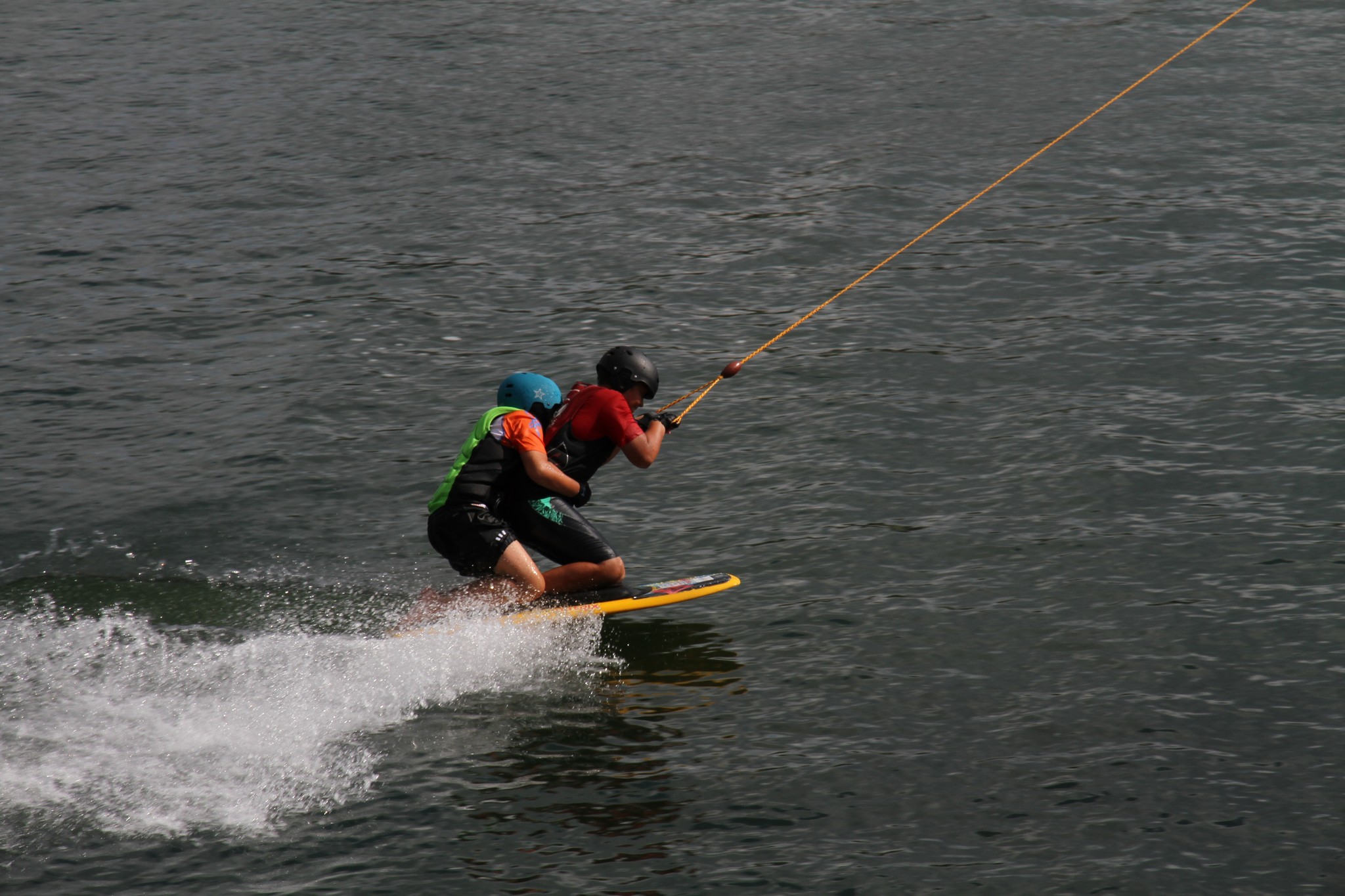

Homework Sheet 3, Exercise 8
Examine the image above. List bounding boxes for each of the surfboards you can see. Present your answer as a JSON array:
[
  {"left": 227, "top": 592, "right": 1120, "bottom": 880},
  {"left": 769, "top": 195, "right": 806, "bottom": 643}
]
[{"left": 387, "top": 571, "right": 743, "bottom": 643}]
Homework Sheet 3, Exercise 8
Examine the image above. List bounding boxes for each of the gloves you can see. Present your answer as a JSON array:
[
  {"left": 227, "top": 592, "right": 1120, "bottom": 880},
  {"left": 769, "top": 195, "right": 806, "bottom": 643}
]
[{"left": 569, "top": 482, "right": 593, "bottom": 508}]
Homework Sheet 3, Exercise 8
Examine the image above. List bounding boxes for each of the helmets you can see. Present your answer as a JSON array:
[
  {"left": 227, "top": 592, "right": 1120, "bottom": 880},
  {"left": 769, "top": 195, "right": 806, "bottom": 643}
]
[
  {"left": 600, "top": 346, "right": 660, "bottom": 401},
  {"left": 497, "top": 372, "right": 563, "bottom": 426}
]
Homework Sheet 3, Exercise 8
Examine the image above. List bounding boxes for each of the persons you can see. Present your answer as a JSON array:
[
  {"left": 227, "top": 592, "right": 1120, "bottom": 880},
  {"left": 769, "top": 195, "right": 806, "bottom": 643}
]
[
  {"left": 402, "top": 370, "right": 593, "bottom": 628},
  {"left": 499, "top": 346, "right": 679, "bottom": 594}
]
